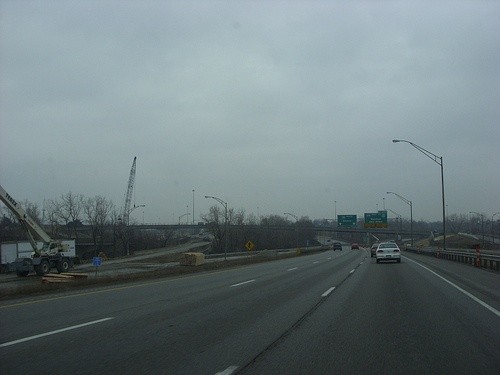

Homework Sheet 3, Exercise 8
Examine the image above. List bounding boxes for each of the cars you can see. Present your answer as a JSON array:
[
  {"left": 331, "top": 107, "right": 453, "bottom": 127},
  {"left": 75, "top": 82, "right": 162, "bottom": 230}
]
[
  {"left": 376, "top": 242, "right": 402, "bottom": 263},
  {"left": 352, "top": 243, "right": 360, "bottom": 250},
  {"left": 371, "top": 243, "right": 379, "bottom": 257}
]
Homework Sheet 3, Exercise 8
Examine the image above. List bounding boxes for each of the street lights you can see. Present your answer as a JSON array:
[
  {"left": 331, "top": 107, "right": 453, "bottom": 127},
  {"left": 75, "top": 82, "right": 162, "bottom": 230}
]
[
  {"left": 178, "top": 213, "right": 191, "bottom": 241},
  {"left": 192, "top": 189, "right": 195, "bottom": 225},
  {"left": 334, "top": 201, "right": 337, "bottom": 219},
  {"left": 386, "top": 191, "right": 414, "bottom": 247},
  {"left": 469, "top": 211, "right": 485, "bottom": 248},
  {"left": 283, "top": 213, "right": 299, "bottom": 257},
  {"left": 384, "top": 208, "right": 403, "bottom": 230},
  {"left": 383, "top": 198, "right": 386, "bottom": 210},
  {"left": 129, "top": 205, "right": 146, "bottom": 214},
  {"left": 393, "top": 139, "right": 446, "bottom": 250},
  {"left": 492, "top": 212, "right": 500, "bottom": 244},
  {"left": 205, "top": 195, "right": 228, "bottom": 260}
]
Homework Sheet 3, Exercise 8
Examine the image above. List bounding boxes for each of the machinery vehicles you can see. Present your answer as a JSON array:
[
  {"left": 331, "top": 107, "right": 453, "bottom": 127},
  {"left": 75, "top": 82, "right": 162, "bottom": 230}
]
[{"left": 0, "top": 186, "right": 74, "bottom": 276}]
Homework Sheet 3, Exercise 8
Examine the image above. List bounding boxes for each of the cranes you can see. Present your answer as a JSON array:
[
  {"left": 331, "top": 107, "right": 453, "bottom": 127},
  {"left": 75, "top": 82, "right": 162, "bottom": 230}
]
[{"left": 123, "top": 157, "right": 137, "bottom": 224}]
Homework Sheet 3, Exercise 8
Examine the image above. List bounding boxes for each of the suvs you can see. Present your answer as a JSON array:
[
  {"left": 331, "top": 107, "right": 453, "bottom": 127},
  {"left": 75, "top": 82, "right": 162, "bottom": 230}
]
[{"left": 333, "top": 242, "right": 343, "bottom": 251}]
